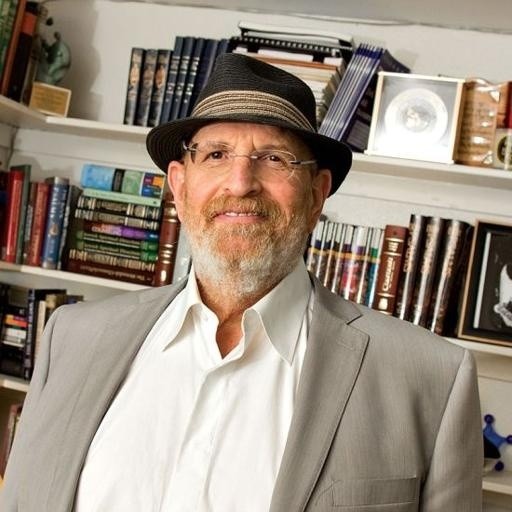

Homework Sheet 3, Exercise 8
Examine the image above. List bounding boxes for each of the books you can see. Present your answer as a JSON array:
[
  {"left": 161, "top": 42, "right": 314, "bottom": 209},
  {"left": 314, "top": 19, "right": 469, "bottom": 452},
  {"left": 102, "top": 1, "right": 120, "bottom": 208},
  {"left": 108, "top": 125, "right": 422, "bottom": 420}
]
[{"left": 0, "top": 403, "right": 23, "bottom": 478}]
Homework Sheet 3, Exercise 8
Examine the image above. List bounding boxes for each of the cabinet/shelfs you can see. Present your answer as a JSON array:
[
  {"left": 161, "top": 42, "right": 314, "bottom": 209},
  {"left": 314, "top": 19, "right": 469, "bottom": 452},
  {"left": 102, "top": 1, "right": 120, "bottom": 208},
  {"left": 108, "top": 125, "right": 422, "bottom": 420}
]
[{"left": 0, "top": 94, "right": 512, "bottom": 498}]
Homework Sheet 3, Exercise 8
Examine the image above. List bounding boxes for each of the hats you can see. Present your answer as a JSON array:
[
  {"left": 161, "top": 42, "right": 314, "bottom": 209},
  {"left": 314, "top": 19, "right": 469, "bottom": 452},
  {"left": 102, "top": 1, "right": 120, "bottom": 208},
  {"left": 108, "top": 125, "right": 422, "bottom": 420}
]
[{"left": 145, "top": 52, "right": 353, "bottom": 198}]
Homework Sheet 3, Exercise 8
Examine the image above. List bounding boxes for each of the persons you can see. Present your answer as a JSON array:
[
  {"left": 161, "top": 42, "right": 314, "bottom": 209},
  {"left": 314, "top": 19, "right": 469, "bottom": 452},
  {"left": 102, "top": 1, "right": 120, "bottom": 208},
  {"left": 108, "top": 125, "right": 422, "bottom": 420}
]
[{"left": 1, "top": 51, "right": 484, "bottom": 512}]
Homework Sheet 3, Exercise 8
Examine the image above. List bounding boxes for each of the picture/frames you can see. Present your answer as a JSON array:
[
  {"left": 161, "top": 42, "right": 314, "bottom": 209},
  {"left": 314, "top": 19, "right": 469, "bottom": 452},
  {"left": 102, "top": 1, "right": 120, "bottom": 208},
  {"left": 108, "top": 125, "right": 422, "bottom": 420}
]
[
  {"left": 363, "top": 71, "right": 468, "bottom": 164},
  {"left": 458, "top": 217, "right": 512, "bottom": 348}
]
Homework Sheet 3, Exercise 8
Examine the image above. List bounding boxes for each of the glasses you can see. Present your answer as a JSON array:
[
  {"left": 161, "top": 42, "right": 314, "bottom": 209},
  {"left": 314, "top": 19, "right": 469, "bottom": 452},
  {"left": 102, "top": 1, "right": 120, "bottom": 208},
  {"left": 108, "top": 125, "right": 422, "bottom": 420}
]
[{"left": 182, "top": 139, "right": 317, "bottom": 183}]
